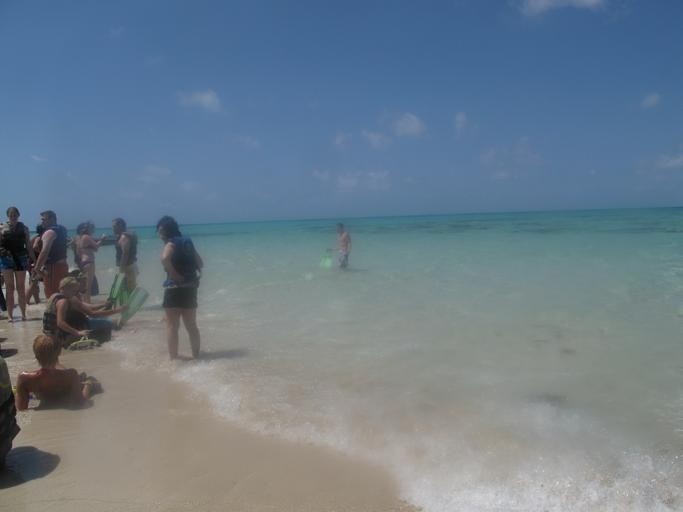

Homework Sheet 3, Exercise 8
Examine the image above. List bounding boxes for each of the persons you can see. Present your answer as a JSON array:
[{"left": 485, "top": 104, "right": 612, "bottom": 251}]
[{"left": 335, "top": 224, "right": 350, "bottom": 268}]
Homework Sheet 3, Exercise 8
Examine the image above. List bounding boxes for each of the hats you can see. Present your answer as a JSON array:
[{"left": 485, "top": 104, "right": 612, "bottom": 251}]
[{"left": 74, "top": 272, "right": 88, "bottom": 279}]
